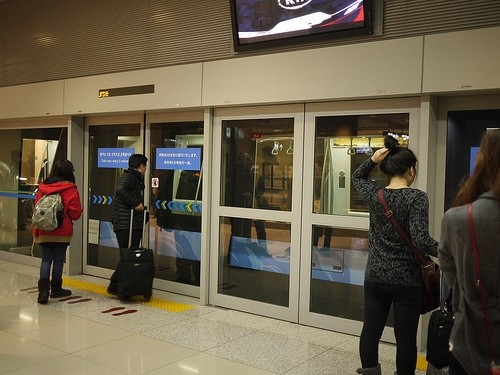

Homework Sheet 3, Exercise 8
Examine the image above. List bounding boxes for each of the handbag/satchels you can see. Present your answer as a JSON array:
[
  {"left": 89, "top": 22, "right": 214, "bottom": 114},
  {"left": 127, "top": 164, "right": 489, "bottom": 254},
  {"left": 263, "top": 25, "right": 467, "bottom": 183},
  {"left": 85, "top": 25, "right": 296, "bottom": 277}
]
[
  {"left": 427, "top": 310, "right": 454, "bottom": 369},
  {"left": 488, "top": 360, "right": 500, "bottom": 375},
  {"left": 424, "top": 255, "right": 440, "bottom": 294}
]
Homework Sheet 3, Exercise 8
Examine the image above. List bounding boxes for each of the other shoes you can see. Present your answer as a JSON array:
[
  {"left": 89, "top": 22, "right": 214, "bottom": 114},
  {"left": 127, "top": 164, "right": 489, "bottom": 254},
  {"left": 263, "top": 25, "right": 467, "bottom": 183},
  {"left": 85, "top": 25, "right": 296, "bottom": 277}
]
[
  {"left": 107, "top": 283, "right": 117, "bottom": 295},
  {"left": 257, "top": 247, "right": 272, "bottom": 258},
  {"left": 286, "top": 247, "right": 290, "bottom": 252},
  {"left": 319, "top": 247, "right": 330, "bottom": 254}
]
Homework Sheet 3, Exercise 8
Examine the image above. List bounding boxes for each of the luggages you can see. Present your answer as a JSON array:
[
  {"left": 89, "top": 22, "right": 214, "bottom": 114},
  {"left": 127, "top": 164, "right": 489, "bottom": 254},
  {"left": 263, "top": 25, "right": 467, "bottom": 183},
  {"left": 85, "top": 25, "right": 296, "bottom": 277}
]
[
  {"left": 117, "top": 206, "right": 154, "bottom": 302},
  {"left": 227, "top": 193, "right": 254, "bottom": 269}
]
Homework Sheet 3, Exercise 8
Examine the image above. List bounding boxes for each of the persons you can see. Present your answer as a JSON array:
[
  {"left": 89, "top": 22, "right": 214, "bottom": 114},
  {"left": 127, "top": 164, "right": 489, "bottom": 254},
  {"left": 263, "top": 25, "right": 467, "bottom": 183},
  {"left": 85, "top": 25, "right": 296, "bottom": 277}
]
[
  {"left": 440, "top": 130, "right": 500, "bottom": 375},
  {"left": 33, "top": 159, "right": 81, "bottom": 303},
  {"left": 157, "top": 145, "right": 332, "bottom": 286},
  {"left": 107, "top": 154, "right": 148, "bottom": 295},
  {"left": 3, "top": 149, "right": 35, "bottom": 233},
  {"left": 352, "top": 136, "right": 439, "bottom": 375}
]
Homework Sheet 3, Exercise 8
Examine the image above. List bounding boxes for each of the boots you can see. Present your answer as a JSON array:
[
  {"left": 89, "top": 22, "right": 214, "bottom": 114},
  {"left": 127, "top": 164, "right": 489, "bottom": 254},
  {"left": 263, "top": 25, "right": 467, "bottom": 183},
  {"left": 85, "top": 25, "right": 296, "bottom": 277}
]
[
  {"left": 38, "top": 279, "right": 49, "bottom": 302},
  {"left": 356, "top": 363, "right": 382, "bottom": 375},
  {"left": 50, "top": 278, "right": 71, "bottom": 298}
]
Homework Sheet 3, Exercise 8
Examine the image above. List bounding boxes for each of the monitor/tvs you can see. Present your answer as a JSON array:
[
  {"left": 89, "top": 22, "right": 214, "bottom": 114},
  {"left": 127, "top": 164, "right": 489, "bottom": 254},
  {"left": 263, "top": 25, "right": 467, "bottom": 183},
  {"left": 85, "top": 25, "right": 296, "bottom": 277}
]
[{"left": 229, "top": 0, "right": 373, "bottom": 52}]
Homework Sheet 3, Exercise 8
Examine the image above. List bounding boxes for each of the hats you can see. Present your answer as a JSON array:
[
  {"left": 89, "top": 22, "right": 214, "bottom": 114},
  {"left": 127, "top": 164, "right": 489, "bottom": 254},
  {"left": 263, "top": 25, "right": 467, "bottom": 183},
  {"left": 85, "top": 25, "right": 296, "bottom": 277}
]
[{"left": 55, "top": 159, "right": 75, "bottom": 171}]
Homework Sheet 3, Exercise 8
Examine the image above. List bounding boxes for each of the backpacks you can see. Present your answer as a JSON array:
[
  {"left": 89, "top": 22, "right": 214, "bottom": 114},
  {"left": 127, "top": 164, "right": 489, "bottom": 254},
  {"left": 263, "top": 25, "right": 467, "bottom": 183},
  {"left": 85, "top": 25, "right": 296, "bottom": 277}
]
[{"left": 31, "top": 193, "right": 64, "bottom": 231}]
